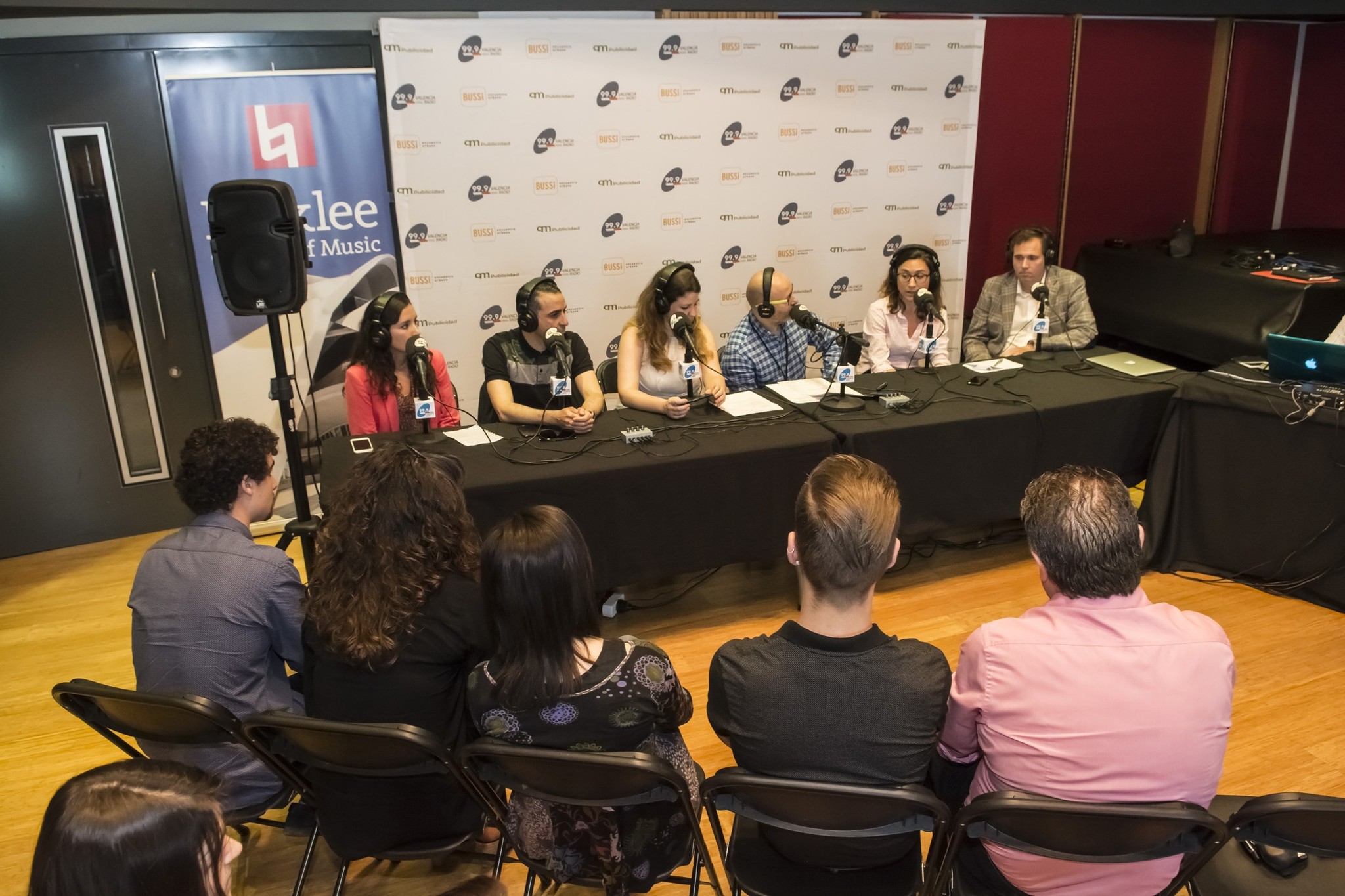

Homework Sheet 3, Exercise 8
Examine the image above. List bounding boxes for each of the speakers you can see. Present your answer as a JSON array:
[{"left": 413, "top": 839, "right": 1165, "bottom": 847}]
[{"left": 207, "top": 178, "right": 312, "bottom": 317}]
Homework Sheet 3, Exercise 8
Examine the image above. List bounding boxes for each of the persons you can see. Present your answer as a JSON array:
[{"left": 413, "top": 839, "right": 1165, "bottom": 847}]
[
  {"left": 931, "top": 467, "right": 1233, "bottom": 895},
  {"left": 27, "top": 759, "right": 243, "bottom": 895},
  {"left": 856, "top": 244, "right": 953, "bottom": 376},
  {"left": 703, "top": 453, "right": 952, "bottom": 896},
  {"left": 616, "top": 262, "right": 726, "bottom": 418},
  {"left": 479, "top": 278, "right": 605, "bottom": 434},
  {"left": 963, "top": 228, "right": 1099, "bottom": 364},
  {"left": 719, "top": 268, "right": 844, "bottom": 396},
  {"left": 123, "top": 419, "right": 323, "bottom": 835},
  {"left": 466, "top": 506, "right": 701, "bottom": 895},
  {"left": 342, "top": 291, "right": 461, "bottom": 436},
  {"left": 298, "top": 440, "right": 512, "bottom": 861}
]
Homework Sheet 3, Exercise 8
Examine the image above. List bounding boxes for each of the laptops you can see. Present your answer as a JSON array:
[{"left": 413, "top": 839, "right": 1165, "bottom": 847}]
[
  {"left": 1086, "top": 351, "right": 1177, "bottom": 377},
  {"left": 1266, "top": 333, "right": 1345, "bottom": 387}
]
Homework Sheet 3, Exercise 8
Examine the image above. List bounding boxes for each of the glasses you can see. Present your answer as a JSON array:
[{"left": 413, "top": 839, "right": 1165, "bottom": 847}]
[
  {"left": 897, "top": 272, "right": 930, "bottom": 284},
  {"left": 755, "top": 283, "right": 793, "bottom": 308}
]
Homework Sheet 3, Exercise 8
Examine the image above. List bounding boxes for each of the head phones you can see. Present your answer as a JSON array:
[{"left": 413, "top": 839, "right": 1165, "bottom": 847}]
[
  {"left": 757, "top": 266, "right": 775, "bottom": 318},
  {"left": 517, "top": 277, "right": 557, "bottom": 333},
  {"left": 369, "top": 291, "right": 400, "bottom": 351},
  {"left": 1005, "top": 226, "right": 1056, "bottom": 265},
  {"left": 654, "top": 262, "right": 695, "bottom": 315},
  {"left": 889, "top": 244, "right": 941, "bottom": 287}
]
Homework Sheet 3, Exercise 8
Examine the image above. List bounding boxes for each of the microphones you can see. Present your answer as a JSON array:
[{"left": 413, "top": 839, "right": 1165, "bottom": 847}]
[
  {"left": 913, "top": 288, "right": 946, "bottom": 324},
  {"left": 405, "top": 335, "right": 429, "bottom": 387},
  {"left": 790, "top": 304, "right": 824, "bottom": 340},
  {"left": 669, "top": 311, "right": 703, "bottom": 364},
  {"left": 1031, "top": 282, "right": 1050, "bottom": 307},
  {"left": 543, "top": 327, "right": 568, "bottom": 378}
]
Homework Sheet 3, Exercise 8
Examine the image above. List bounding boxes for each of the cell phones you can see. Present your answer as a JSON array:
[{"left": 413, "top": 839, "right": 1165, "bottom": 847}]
[
  {"left": 687, "top": 394, "right": 712, "bottom": 404},
  {"left": 966, "top": 376, "right": 989, "bottom": 386},
  {"left": 516, "top": 424, "right": 542, "bottom": 438},
  {"left": 350, "top": 437, "right": 373, "bottom": 453},
  {"left": 1063, "top": 363, "right": 1094, "bottom": 370}
]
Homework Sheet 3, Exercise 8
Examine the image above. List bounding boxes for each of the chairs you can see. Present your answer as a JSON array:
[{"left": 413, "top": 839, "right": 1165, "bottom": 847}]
[
  {"left": 244, "top": 708, "right": 552, "bottom": 896},
  {"left": 459, "top": 733, "right": 725, "bottom": 896},
  {"left": 50, "top": 676, "right": 323, "bottom": 895},
  {"left": 933, "top": 788, "right": 1230, "bottom": 896},
  {"left": 697, "top": 764, "right": 952, "bottom": 896},
  {"left": 1184, "top": 793, "right": 1345, "bottom": 896}
]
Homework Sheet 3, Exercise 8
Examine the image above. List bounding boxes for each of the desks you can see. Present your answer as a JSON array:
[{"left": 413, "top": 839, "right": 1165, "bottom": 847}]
[
  {"left": 1134, "top": 356, "right": 1345, "bottom": 614},
  {"left": 316, "top": 386, "right": 843, "bottom": 589},
  {"left": 758, "top": 336, "right": 1198, "bottom": 549}
]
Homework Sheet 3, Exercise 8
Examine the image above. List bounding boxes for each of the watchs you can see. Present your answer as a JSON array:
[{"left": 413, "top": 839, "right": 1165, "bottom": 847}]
[{"left": 584, "top": 408, "right": 596, "bottom": 422}]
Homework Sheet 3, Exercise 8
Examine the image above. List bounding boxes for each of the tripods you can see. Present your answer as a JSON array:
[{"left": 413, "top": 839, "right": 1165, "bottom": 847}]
[{"left": 268, "top": 314, "right": 323, "bottom": 600}]
